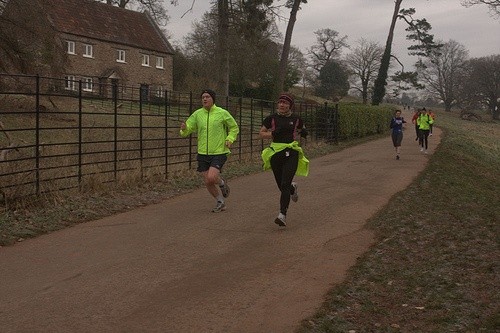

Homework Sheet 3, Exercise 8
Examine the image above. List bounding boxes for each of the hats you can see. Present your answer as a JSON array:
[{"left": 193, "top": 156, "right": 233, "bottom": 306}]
[
  {"left": 201, "top": 89, "right": 216, "bottom": 103},
  {"left": 278, "top": 93, "right": 294, "bottom": 106}
]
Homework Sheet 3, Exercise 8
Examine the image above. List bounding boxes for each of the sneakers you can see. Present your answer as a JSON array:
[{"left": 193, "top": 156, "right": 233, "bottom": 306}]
[
  {"left": 291, "top": 181, "right": 298, "bottom": 203},
  {"left": 212, "top": 201, "right": 226, "bottom": 212},
  {"left": 274, "top": 216, "right": 287, "bottom": 226},
  {"left": 219, "top": 176, "right": 230, "bottom": 198}
]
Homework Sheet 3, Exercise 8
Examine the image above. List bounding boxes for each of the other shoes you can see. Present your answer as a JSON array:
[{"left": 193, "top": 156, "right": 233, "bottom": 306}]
[
  {"left": 396, "top": 154, "right": 400, "bottom": 160},
  {"left": 420, "top": 148, "right": 429, "bottom": 153}
]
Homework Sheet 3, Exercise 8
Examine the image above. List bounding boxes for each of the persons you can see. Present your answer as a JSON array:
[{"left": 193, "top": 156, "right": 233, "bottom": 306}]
[
  {"left": 179, "top": 90, "right": 240, "bottom": 213},
  {"left": 390, "top": 109, "right": 407, "bottom": 160},
  {"left": 412, "top": 108, "right": 437, "bottom": 149},
  {"left": 259, "top": 92, "right": 310, "bottom": 227}
]
